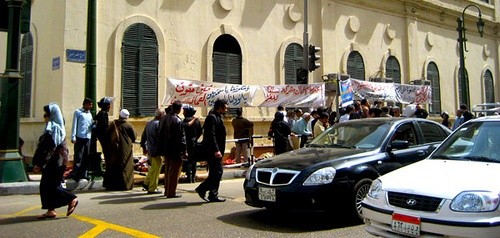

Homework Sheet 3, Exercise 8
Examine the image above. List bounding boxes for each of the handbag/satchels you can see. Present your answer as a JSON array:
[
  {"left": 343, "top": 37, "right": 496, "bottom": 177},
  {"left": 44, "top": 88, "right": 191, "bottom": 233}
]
[{"left": 193, "top": 142, "right": 206, "bottom": 161}]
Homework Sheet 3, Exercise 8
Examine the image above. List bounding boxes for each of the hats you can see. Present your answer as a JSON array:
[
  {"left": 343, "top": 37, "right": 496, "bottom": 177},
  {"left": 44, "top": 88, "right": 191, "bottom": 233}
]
[
  {"left": 120, "top": 109, "right": 130, "bottom": 118},
  {"left": 104, "top": 96, "right": 115, "bottom": 104}
]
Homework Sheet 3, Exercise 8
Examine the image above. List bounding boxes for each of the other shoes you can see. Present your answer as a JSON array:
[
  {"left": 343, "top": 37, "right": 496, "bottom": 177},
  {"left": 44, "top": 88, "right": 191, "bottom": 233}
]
[
  {"left": 142, "top": 184, "right": 162, "bottom": 194},
  {"left": 195, "top": 188, "right": 210, "bottom": 202},
  {"left": 168, "top": 194, "right": 182, "bottom": 198},
  {"left": 183, "top": 179, "right": 195, "bottom": 183},
  {"left": 209, "top": 197, "right": 226, "bottom": 202}
]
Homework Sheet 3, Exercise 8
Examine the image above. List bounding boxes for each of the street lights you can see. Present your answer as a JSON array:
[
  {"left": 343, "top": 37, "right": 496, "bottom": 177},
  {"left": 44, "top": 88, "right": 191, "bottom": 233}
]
[{"left": 453, "top": 3, "right": 485, "bottom": 114}]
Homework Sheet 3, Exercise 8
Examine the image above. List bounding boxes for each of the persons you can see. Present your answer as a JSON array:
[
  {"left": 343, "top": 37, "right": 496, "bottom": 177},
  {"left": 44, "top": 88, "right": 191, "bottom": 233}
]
[
  {"left": 195, "top": 100, "right": 228, "bottom": 203},
  {"left": 267, "top": 98, "right": 429, "bottom": 155},
  {"left": 231, "top": 108, "right": 251, "bottom": 168},
  {"left": 32, "top": 103, "right": 78, "bottom": 218},
  {"left": 92, "top": 97, "right": 137, "bottom": 191},
  {"left": 71, "top": 98, "right": 95, "bottom": 182},
  {"left": 441, "top": 104, "right": 486, "bottom": 132},
  {"left": 140, "top": 101, "right": 203, "bottom": 198}
]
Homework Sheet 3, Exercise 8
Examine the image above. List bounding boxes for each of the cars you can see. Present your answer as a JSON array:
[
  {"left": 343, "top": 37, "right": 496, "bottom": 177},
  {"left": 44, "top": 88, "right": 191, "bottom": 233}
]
[
  {"left": 240, "top": 117, "right": 472, "bottom": 223},
  {"left": 361, "top": 116, "right": 500, "bottom": 237}
]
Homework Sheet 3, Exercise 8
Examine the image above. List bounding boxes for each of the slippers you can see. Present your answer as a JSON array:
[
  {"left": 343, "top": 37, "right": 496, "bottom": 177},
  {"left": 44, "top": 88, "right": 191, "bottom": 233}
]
[
  {"left": 67, "top": 200, "right": 78, "bottom": 216},
  {"left": 37, "top": 214, "right": 56, "bottom": 219}
]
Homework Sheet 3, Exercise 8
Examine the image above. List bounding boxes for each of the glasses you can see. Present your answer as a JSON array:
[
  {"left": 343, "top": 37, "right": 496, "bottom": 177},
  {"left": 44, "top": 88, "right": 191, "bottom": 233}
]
[{"left": 44, "top": 113, "right": 48, "bottom": 118}]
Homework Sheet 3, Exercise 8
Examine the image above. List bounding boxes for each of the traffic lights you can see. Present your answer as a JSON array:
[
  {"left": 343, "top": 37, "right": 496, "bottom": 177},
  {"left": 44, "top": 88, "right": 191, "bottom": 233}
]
[{"left": 309, "top": 44, "right": 322, "bottom": 71}]
